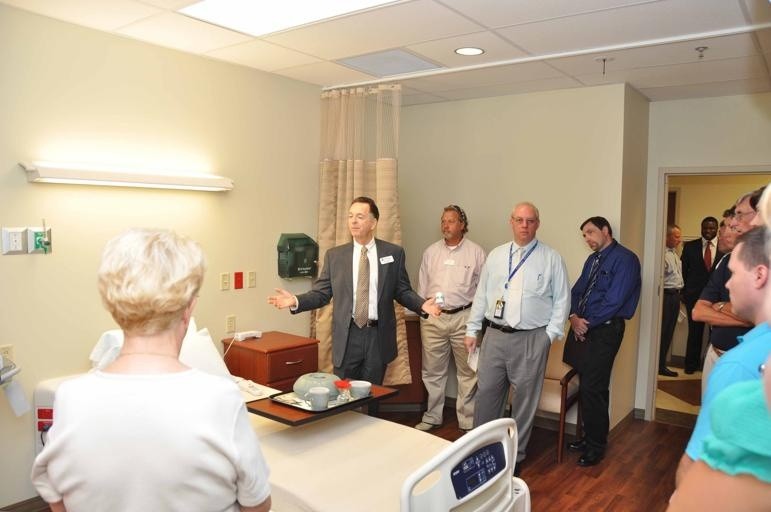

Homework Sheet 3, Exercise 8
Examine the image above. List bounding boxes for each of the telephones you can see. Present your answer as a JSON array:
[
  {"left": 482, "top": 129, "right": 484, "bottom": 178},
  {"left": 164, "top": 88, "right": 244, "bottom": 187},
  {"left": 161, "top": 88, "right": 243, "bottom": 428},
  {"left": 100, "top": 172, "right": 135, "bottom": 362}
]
[{"left": 235, "top": 330, "right": 262, "bottom": 341}]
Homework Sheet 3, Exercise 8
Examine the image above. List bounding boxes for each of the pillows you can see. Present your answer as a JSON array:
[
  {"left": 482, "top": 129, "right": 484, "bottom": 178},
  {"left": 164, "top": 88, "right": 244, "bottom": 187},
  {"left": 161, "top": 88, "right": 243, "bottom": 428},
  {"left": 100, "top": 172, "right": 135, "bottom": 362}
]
[
  {"left": 100, "top": 327, "right": 236, "bottom": 384},
  {"left": 89, "top": 317, "right": 196, "bottom": 367}
]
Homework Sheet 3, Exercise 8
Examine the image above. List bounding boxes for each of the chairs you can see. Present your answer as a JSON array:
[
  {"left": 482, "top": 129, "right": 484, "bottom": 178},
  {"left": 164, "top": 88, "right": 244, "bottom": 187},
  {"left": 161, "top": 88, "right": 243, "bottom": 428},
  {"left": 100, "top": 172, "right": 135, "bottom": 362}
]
[{"left": 505, "top": 320, "right": 582, "bottom": 463}]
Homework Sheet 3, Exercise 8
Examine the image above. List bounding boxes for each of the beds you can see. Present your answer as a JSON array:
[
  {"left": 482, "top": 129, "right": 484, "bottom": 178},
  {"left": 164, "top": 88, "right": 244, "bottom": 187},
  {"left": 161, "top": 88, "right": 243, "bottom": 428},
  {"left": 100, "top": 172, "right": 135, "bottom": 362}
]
[{"left": 34, "top": 373, "right": 532, "bottom": 512}]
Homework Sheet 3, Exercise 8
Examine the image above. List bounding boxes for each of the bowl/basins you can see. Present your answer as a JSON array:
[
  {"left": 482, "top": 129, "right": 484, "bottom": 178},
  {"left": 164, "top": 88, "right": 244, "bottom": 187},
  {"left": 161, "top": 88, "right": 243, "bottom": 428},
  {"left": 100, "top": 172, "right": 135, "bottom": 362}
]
[
  {"left": 294, "top": 373, "right": 342, "bottom": 402},
  {"left": 350, "top": 380, "right": 373, "bottom": 398}
]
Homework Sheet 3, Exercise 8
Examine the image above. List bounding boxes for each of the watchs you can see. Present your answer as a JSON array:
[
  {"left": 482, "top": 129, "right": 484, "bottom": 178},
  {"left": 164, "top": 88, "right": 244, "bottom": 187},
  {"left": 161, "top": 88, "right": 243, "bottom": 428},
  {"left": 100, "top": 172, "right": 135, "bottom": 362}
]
[{"left": 568, "top": 312, "right": 576, "bottom": 320}]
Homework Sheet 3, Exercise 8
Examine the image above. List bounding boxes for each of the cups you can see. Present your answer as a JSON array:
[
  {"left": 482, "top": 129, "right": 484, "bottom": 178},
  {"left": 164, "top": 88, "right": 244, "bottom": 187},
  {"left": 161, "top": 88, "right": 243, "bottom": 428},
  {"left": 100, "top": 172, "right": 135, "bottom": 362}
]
[{"left": 304, "top": 386, "right": 330, "bottom": 412}]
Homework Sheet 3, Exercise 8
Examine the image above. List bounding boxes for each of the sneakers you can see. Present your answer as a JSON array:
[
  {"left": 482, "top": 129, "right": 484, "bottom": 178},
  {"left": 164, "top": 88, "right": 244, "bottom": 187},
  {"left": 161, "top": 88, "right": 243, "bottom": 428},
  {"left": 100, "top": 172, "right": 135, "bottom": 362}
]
[{"left": 415, "top": 422, "right": 441, "bottom": 431}]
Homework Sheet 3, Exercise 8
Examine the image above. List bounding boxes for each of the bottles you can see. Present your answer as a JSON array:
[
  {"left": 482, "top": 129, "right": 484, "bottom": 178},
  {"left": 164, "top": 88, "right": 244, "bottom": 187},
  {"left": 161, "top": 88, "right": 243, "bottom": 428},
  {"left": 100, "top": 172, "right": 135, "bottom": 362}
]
[{"left": 336, "top": 379, "right": 350, "bottom": 403}]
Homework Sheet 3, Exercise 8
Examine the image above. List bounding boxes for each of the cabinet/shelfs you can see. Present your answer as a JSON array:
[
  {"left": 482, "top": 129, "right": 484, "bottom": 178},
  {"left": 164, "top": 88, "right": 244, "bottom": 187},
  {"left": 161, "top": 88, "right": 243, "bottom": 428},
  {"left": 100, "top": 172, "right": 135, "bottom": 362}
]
[{"left": 220, "top": 331, "right": 320, "bottom": 393}]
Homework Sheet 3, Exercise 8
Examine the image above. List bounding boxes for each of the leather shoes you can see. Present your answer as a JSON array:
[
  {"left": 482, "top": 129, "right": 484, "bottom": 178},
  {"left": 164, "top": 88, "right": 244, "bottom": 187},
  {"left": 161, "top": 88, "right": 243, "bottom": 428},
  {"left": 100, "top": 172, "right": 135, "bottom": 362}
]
[
  {"left": 578, "top": 447, "right": 606, "bottom": 465},
  {"left": 568, "top": 440, "right": 587, "bottom": 451},
  {"left": 659, "top": 368, "right": 677, "bottom": 376},
  {"left": 685, "top": 366, "right": 695, "bottom": 374}
]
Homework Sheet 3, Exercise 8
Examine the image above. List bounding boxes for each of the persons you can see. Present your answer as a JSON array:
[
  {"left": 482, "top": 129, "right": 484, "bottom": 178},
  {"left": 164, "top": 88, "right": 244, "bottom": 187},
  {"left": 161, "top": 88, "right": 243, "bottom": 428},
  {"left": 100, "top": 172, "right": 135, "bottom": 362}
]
[
  {"left": 267, "top": 197, "right": 443, "bottom": 417},
  {"left": 415, "top": 204, "right": 489, "bottom": 432},
  {"left": 659, "top": 183, "right": 771, "bottom": 511},
  {"left": 463, "top": 202, "right": 573, "bottom": 477},
  {"left": 567, "top": 216, "right": 641, "bottom": 467},
  {"left": 30, "top": 228, "right": 272, "bottom": 512}
]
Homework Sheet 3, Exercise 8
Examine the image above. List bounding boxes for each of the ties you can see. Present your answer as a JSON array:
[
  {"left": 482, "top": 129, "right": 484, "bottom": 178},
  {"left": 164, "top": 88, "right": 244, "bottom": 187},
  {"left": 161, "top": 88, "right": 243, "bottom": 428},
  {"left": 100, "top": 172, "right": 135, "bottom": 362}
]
[
  {"left": 505, "top": 248, "right": 524, "bottom": 328},
  {"left": 705, "top": 242, "right": 711, "bottom": 270},
  {"left": 577, "top": 253, "right": 602, "bottom": 316},
  {"left": 354, "top": 246, "right": 369, "bottom": 328}
]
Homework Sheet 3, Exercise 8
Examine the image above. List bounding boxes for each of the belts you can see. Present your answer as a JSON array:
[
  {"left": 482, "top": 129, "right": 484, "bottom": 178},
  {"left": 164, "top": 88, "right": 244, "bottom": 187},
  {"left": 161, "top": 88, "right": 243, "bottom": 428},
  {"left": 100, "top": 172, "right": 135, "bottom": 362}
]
[
  {"left": 366, "top": 320, "right": 378, "bottom": 327},
  {"left": 485, "top": 320, "right": 517, "bottom": 333},
  {"left": 440, "top": 303, "right": 472, "bottom": 314}
]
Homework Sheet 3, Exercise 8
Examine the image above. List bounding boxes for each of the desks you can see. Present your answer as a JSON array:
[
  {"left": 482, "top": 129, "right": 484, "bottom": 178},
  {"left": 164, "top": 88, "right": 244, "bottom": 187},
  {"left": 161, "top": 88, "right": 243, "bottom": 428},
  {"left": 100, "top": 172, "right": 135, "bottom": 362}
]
[{"left": 380, "top": 301, "right": 423, "bottom": 412}]
